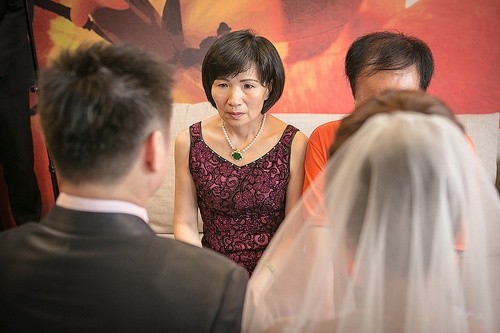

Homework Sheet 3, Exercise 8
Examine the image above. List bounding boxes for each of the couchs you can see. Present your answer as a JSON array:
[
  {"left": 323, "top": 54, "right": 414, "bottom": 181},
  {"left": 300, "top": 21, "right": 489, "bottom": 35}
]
[{"left": 142, "top": 101, "right": 500, "bottom": 249}]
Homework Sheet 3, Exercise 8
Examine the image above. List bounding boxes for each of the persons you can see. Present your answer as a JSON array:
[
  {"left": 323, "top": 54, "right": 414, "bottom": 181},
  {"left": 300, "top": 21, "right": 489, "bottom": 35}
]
[
  {"left": 241, "top": 89, "right": 500, "bottom": 333},
  {"left": 0, "top": 40, "right": 249, "bottom": 333},
  {"left": 302, "top": 30, "right": 475, "bottom": 276},
  {"left": 173, "top": 27, "right": 308, "bottom": 277}
]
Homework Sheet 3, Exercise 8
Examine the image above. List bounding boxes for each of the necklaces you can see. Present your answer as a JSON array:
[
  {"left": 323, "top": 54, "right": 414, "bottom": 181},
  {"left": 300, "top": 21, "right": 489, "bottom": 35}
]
[{"left": 220, "top": 112, "right": 266, "bottom": 161}]
